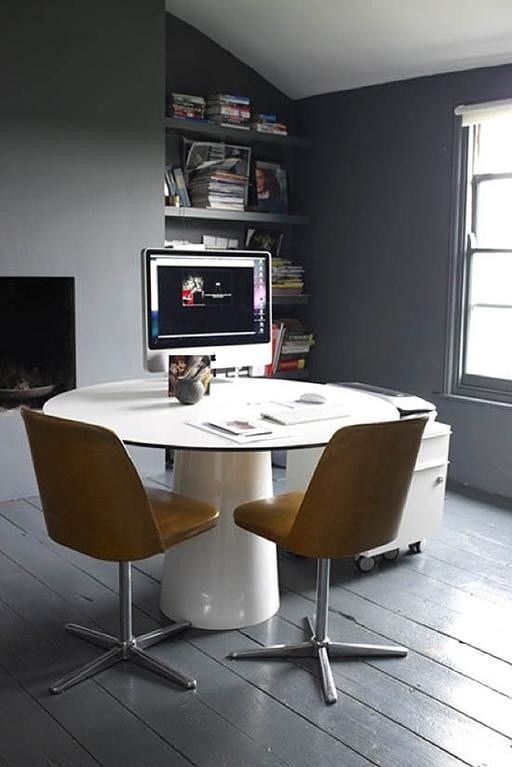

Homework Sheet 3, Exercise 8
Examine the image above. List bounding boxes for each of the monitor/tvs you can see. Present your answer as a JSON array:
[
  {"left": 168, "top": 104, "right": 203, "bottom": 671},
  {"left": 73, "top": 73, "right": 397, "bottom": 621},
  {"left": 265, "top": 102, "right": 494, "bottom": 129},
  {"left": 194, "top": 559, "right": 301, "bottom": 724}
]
[
  {"left": 141, "top": 247, "right": 272, "bottom": 373},
  {"left": 1, "top": 277, "right": 77, "bottom": 409}
]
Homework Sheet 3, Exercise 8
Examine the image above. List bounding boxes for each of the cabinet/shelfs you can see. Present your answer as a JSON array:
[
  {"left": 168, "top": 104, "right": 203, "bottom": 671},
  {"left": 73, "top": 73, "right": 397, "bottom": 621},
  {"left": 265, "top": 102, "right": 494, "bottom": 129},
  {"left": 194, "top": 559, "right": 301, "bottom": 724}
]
[
  {"left": 163, "top": 92, "right": 312, "bottom": 383},
  {"left": 281, "top": 421, "right": 453, "bottom": 572}
]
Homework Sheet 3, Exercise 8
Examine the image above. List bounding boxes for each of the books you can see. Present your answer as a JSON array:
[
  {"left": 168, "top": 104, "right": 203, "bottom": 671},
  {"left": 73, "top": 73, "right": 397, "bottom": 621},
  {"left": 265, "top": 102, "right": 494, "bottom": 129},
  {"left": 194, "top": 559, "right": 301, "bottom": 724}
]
[
  {"left": 167, "top": 91, "right": 288, "bottom": 213},
  {"left": 246, "top": 227, "right": 305, "bottom": 295},
  {"left": 264, "top": 317, "right": 316, "bottom": 378}
]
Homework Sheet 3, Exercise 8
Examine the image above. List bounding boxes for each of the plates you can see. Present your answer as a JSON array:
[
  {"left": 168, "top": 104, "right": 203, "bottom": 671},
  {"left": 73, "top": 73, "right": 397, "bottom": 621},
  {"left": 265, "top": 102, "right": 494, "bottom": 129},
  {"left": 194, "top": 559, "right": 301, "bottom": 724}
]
[{"left": 1, "top": 385, "right": 56, "bottom": 401}]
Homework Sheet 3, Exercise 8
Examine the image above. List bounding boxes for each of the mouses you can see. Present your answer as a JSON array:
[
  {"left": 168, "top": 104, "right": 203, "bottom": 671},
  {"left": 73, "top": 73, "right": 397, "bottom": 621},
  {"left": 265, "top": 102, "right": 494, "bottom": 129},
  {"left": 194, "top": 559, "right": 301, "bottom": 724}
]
[{"left": 300, "top": 393, "right": 326, "bottom": 404}]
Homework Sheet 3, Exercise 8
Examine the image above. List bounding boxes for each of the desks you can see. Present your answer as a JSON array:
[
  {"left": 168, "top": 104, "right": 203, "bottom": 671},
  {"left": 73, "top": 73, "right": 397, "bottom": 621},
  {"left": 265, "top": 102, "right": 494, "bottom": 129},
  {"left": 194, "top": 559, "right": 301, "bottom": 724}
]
[{"left": 42, "top": 374, "right": 401, "bottom": 631}]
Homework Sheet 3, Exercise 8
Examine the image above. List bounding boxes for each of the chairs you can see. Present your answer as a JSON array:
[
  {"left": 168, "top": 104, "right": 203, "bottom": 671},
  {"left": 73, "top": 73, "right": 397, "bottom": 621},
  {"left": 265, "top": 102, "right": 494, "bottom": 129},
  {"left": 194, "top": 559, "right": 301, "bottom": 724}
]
[
  {"left": 228, "top": 415, "right": 430, "bottom": 706},
  {"left": 19, "top": 406, "right": 219, "bottom": 696}
]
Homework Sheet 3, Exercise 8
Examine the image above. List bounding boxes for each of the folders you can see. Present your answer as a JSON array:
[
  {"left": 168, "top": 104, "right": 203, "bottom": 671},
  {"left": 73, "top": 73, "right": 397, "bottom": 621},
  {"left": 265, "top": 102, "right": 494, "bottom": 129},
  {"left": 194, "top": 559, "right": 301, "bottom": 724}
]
[{"left": 173, "top": 167, "right": 191, "bottom": 207}]
